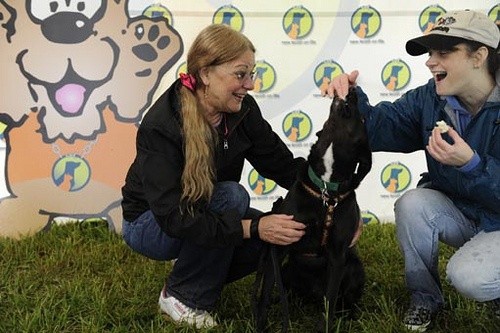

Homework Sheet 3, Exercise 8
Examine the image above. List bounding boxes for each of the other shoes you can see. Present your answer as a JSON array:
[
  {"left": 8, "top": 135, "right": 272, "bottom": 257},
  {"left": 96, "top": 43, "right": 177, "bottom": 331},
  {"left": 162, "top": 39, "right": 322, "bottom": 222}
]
[{"left": 401, "top": 304, "right": 433, "bottom": 331}]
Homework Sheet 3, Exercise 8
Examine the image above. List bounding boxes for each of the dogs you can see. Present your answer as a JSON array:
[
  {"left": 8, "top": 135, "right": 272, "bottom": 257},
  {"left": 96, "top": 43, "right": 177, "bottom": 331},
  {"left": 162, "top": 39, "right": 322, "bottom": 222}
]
[{"left": 243, "top": 84, "right": 373, "bottom": 333}]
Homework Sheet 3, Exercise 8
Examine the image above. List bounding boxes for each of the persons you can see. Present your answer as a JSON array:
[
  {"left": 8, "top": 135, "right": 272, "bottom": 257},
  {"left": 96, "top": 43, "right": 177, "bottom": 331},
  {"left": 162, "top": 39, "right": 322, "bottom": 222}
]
[
  {"left": 319, "top": 8, "right": 500, "bottom": 333},
  {"left": 120, "top": 22, "right": 364, "bottom": 333}
]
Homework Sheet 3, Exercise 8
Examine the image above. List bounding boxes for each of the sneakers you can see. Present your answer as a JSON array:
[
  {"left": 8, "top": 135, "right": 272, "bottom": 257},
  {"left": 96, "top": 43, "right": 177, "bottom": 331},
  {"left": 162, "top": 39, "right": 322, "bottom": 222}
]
[{"left": 158, "top": 283, "right": 218, "bottom": 329}]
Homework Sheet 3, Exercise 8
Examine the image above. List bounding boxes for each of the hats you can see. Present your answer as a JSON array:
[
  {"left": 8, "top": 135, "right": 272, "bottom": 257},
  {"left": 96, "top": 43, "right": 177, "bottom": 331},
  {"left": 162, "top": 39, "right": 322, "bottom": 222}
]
[{"left": 405, "top": 8, "right": 500, "bottom": 56}]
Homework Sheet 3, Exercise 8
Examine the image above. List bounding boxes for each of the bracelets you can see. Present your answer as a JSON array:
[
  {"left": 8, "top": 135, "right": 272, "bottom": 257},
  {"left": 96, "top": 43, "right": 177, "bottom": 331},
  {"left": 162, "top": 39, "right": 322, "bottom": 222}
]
[{"left": 249, "top": 217, "right": 261, "bottom": 241}]
